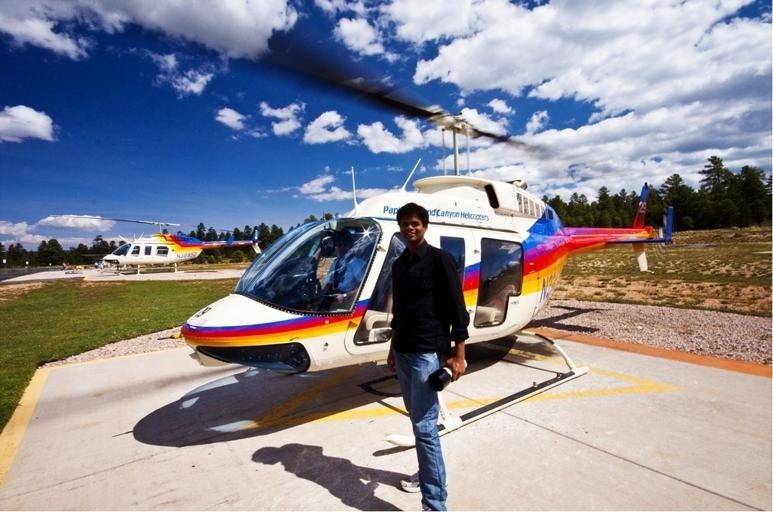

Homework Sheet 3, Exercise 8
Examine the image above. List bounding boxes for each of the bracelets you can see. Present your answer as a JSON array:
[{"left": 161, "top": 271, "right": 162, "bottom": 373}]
[{"left": 343, "top": 293, "right": 348, "bottom": 301}]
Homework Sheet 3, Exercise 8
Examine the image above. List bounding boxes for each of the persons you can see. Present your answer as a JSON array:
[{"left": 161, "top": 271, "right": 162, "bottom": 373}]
[
  {"left": 322, "top": 247, "right": 366, "bottom": 305},
  {"left": 387, "top": 203, "right": 470, "bottom": 511}
]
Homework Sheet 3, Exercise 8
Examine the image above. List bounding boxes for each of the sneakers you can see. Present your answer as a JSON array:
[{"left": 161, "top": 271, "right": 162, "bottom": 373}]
[{"left": 399, "top": 471, "right": 448, "bottom": 493}]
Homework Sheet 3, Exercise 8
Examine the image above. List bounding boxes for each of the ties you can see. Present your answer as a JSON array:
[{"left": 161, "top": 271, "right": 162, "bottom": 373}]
[{"left": 333, "top": 258, "right": 340, "bottom": 289}]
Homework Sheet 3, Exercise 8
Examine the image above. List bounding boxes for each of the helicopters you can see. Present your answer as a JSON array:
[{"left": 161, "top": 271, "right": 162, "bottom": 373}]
[
  {"left": 176, "top": 57, "right": 679, "bottom": 451},
  {"left": 72, "top": 215, "right": 263, "bottom": 276}
]
